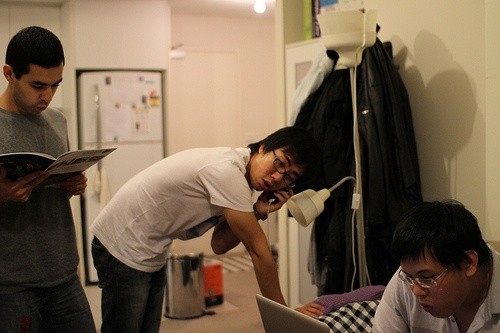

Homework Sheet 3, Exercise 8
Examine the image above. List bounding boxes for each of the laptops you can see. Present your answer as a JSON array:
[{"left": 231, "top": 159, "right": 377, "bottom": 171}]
[{"left": 254, "top": 293, "right": 331, "bottom": 333}]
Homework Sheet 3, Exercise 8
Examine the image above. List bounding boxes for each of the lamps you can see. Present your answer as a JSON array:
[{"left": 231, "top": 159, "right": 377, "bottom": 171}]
[{"left": 286, "top": 9, "right": 376, "bottom": 288}]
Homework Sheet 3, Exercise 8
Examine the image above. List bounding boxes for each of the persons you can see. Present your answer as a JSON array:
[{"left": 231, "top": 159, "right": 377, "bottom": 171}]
[
  {"left": 0, "top": 25, "right": 101, "bottom": 333},
  {"left": 371, "top": 200, "right": 500, "bottom": 333},
  {"left": 90, "top": 126, "right": 325, "bottom": 332}
]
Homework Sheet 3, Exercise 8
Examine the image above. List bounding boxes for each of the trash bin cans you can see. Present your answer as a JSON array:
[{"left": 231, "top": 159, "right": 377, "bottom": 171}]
[{"left": 163, "top": 251, "right": 206, "bottom": 319}]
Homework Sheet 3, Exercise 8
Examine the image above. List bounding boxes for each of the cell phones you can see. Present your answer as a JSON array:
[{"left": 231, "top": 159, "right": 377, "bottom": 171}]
[{"left": 269, "top": 189, "right": 290, "bottom": 203}]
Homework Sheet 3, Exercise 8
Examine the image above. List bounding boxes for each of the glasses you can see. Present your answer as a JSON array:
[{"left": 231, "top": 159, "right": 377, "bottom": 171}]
[
  {"left": 272, "top": 150, "right": 295, "bottom": 188},
  {"left": 398, "top": 264, "right": 452, "bottom": 288}
]
